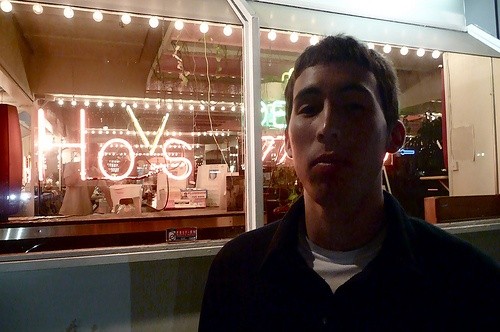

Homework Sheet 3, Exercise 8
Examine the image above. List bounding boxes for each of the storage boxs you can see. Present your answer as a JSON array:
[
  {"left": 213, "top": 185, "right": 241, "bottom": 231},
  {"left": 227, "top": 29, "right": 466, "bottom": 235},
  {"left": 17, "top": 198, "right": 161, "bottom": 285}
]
[
  {"left": 195, "top": 163, "right": 227, "bottom": 208},
  {"left": 158, "top": 187, "right": 207, "bottom": 209},
  {"left": 155, "top": 169, "right": 186, "bottom": 209}
]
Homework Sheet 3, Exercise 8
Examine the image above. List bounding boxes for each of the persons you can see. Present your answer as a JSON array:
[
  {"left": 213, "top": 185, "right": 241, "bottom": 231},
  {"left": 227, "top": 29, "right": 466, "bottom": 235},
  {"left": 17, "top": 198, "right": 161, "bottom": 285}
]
[{"left": 192, "top": 32, "right": 499, "bottom": 331}]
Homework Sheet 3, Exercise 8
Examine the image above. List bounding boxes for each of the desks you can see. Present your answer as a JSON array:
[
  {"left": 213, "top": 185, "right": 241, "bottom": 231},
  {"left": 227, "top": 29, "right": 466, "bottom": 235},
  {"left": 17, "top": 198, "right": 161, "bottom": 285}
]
[{"left": 108, "top": 184, "right": 143, "bottom": 213}]
